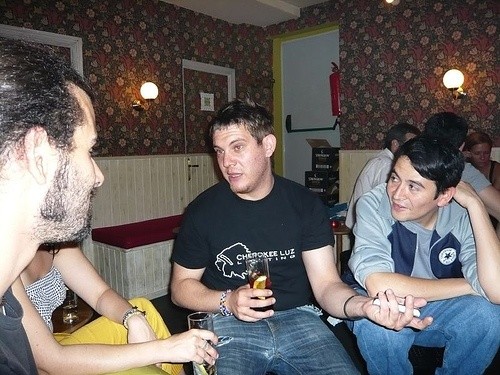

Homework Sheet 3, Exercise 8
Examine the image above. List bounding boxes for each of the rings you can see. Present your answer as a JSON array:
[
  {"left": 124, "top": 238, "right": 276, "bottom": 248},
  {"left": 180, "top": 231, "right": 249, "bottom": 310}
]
[{"left": 201, "top": 341, "right": 207, "bottom": 348}]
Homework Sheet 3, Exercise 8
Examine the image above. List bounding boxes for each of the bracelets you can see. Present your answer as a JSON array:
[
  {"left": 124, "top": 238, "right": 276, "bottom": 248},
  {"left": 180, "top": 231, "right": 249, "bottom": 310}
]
[
  {"left": 343, "top": 294, "right": 363, "bottom": 321},
  {"left": 219, "top": 289, "right": 233, "bottom": 317},
  {"left": 123, "top": 305, "right": 146, "bottom": 330}
]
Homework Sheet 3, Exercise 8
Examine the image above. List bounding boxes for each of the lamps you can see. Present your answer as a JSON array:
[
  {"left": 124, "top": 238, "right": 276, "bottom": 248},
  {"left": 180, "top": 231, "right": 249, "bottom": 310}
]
[
  {"left": 443, "top": 68, "right": 467, "bottom": 99},
  {"left": 131, "top": 82, "right": 159, "bottom": 112}
]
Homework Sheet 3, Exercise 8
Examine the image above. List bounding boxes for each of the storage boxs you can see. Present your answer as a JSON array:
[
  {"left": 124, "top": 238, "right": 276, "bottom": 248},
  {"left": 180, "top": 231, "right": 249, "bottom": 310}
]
[{"left": 304, "top": 138, "right": 342, "bottom": 205}]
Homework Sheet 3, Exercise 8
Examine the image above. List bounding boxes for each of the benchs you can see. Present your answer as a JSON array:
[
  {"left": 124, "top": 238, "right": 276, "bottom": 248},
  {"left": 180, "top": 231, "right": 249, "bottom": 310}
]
[{"left": 91, "top": 214, "right": 183, "bottom": 302}]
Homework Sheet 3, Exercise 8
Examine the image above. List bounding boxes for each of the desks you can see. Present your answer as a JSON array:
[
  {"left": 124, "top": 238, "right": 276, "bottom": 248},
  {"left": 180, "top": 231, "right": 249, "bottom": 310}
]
[
  {"left": 51, "top": 288, "right": 94, "bottom": 335},
  {"left": 332, "top": 222, "right": 352, "bottom": 277}
]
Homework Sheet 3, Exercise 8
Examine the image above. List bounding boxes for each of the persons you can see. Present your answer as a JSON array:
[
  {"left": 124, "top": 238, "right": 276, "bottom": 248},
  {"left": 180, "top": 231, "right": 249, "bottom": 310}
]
[
  {"left": 345, "top": 123, "right": 422, "bottom": 229},
  {"left": 0, "top": 37, "right": 105, "bottom": 375},
  {"left": 10, "top": 241, "right": 219, "bottom": 375},
  {"left": 169, "top": 97, "right": 433, "bottom": 375},
  {"left": 344, "top": 134, "right": 500, "bottom": 375},
  {"left": 424, "top": 112, "right": 500, "bottom": 221}
]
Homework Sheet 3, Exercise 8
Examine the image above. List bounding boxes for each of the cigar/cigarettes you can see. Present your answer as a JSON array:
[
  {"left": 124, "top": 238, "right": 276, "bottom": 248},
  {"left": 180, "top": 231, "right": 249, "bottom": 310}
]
[{"left": 373, "top": 298, "right": 420, "bottom": 318}]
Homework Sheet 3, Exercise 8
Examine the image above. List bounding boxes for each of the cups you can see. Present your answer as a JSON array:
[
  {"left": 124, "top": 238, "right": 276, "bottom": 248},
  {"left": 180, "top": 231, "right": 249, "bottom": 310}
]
[
  {"left": 187, "top": 312, "right": 218, "bottom": 375},
  {"left": 62, "top": 287, "right": 79, "bottom": 325},
  {"left": 245, "top": 257, "right": 272, "bottom": 313}
]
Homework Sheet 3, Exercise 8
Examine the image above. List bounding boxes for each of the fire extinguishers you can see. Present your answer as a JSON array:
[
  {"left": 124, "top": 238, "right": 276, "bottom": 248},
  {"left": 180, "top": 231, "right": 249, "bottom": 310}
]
[{"left": 330, "top": 62, "right": 341, "bottom": 116}]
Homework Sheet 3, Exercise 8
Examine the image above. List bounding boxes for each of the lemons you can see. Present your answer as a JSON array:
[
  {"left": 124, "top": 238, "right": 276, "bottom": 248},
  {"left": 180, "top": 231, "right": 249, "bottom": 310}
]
[{"left": 253, "top": 275, "right": 266, "bottom": 300}]
